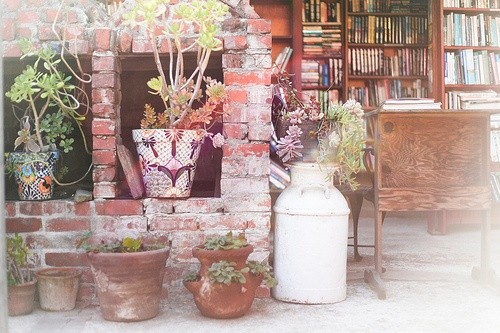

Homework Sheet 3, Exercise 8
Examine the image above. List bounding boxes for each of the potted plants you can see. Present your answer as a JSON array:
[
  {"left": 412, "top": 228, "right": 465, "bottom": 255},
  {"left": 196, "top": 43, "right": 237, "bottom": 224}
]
[
  {"left": 5, "top": 38, "right": 86, "bottom": 201},
  {"left": 6, "top": 232, "right": 39, "bottom": 317},
  {"left": 121, "top": 0, "right": 232, "bottom": 200},
  {"left": 74, "top": 228, "right": 172, "bottom": 323},
  {"left": 272, "top": 64, "right": 367, "bottom": 192},
  {"left": 184, "top": 231, "right": 278, "bottom": 319}
]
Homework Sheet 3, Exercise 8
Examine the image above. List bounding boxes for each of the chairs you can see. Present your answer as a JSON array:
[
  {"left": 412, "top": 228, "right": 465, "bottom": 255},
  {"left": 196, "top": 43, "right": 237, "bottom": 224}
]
[{"left": 327, "top": 172, "right": 388, "bottom": 274}]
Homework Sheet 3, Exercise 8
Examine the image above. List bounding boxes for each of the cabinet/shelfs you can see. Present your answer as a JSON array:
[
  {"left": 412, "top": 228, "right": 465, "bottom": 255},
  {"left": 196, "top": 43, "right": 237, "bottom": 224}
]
[{"left": 249, "top": 0, "right": 500, "bottom": 237}]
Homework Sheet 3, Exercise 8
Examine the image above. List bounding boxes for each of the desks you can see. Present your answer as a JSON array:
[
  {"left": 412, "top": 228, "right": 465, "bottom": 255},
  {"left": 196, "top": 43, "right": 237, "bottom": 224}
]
[{"left": 361, "top": 110, "right": 491, "bottom": 301}]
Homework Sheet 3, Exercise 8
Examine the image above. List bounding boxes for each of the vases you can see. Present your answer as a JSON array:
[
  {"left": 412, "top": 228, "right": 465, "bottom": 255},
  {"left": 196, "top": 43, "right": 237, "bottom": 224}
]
[{"left": 35, "top": 268, "right": 83, "bottom": 312}]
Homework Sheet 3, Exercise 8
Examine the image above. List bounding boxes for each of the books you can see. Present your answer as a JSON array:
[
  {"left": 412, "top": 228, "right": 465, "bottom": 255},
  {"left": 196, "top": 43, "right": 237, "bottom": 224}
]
[{"left": 296, "top": 1, "right": 500, "bottom": 200}]
